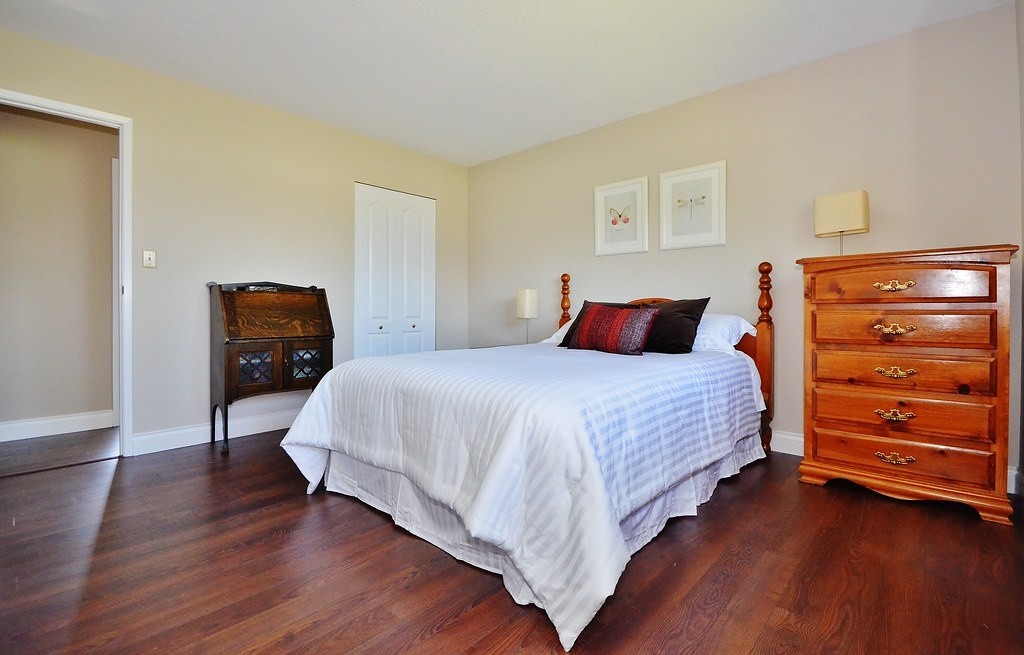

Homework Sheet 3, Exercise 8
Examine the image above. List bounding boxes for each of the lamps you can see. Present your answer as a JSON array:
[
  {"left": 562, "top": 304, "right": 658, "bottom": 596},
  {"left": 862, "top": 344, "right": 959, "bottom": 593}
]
[
  {"left": 812, "top": 188, "right": 871, "bottom": 257},
  {"left": 516, "top": 290, "right": 538, "bottom": 344}
]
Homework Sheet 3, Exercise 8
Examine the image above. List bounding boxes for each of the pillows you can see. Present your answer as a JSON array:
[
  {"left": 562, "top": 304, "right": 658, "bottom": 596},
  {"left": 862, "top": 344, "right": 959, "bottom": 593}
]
[{"left": 539, "top": 296, "right": 759, "bottom": 357}]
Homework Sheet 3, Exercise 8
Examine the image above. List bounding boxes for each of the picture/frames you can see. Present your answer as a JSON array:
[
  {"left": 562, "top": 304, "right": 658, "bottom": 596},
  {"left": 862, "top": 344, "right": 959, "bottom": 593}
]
[
  {"left": 592, "top": 175, "right": 650, "bottom": 257},
  {"left": 658, "top": 158, "right": 730, "bottom": 250}
]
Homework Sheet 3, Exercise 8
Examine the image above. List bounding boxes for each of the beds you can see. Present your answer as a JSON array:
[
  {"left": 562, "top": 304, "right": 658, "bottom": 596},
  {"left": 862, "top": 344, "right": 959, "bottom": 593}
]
[{"left": 279, "top": 260, "right": 774, "bottom": 651}]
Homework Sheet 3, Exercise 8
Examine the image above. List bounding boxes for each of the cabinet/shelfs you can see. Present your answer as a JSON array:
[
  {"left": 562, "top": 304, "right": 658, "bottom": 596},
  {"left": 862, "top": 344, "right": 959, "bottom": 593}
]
[
  {"left": 208, "top": 282, "right": 336, "bottom": 456},
  {"left": 796, "top": 244, "right": 1019, "bottom": 526}
]
